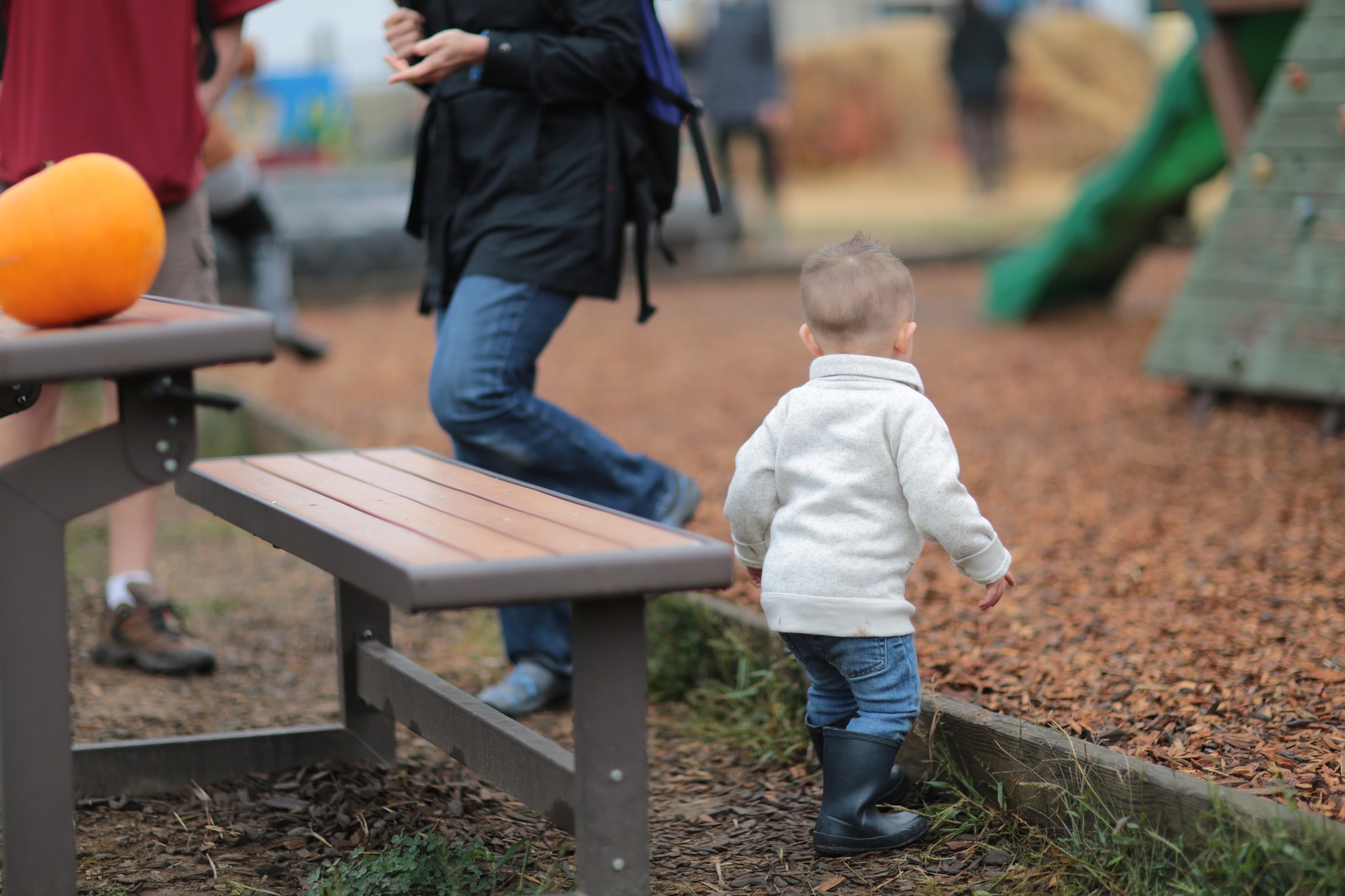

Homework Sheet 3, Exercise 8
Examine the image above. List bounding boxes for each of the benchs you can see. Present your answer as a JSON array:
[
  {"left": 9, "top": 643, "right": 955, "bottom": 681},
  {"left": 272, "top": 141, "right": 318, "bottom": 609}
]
[{"left": 167, "top": 442, "right": 739, "bottom": 895}]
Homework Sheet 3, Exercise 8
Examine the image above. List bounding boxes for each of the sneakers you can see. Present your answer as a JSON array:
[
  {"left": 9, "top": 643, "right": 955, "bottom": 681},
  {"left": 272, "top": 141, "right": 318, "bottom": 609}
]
[
  {"left": 470, "top": 661, "right": 569, "bottom": 717},
  {"left": 88, "top": 580, "right": 217, "bottom": 675}
]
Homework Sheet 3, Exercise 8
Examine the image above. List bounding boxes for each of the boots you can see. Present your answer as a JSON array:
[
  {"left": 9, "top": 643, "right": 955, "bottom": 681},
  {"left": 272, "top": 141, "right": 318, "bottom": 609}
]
[
  {"left": 810, "top": 719, "right": 906, "bottom": 806},
  {"left": 812, "top": 724, "right": 928, "bottom": 855}
]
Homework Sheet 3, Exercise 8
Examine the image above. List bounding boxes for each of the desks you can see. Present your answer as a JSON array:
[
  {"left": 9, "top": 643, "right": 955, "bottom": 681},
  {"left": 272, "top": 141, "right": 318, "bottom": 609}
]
[{"left": 0, "top": 292, "right": 278, "bottom": 896}]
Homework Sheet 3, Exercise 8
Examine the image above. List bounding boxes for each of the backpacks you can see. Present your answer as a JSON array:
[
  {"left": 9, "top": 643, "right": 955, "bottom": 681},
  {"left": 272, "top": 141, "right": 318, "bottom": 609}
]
[{"left": 632, "top": 1, "right": 696, "bottom": 225}]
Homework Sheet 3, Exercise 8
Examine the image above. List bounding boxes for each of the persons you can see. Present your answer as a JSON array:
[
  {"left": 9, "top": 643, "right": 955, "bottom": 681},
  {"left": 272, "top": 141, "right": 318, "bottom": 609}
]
[
  {"left": 192, "top": 41, "right": 331, "bottom": 366},
  {"left": 381, "top": 0, "right": 706, "bottom": 721},
  {"left": 719, "top": 231, "right": 1018, "bottom": 857},
  {"left": 690, "top": 3, "right": 792, "bottom": 247},
  {"left": 1, "top": 0, "right": 274, "bottom": 683},
  {"left": 943, "top": 2, "right": 1014, "bottom": 195}
]
